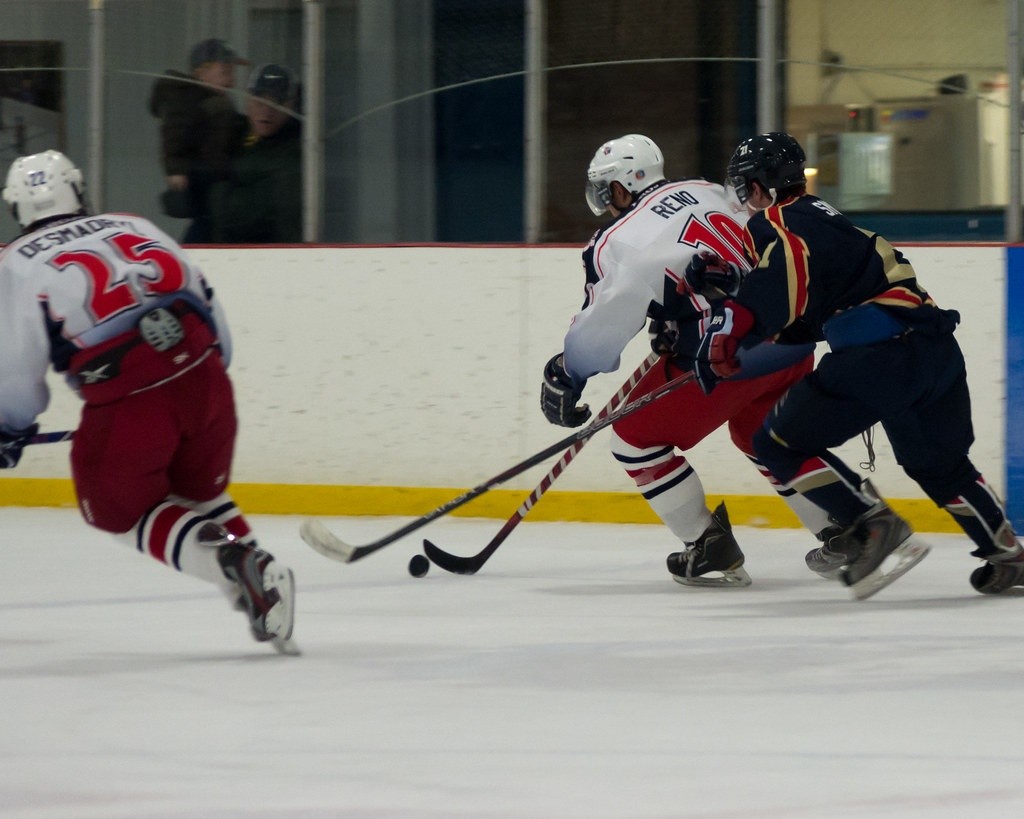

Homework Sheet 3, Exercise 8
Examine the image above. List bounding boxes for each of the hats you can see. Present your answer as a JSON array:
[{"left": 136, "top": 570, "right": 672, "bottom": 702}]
[
  {"left": 189, "top": 38, "right": 250, "bottom": 70},
  {"left": 246, "top": 62, "right": 291, "bottom": 104}
]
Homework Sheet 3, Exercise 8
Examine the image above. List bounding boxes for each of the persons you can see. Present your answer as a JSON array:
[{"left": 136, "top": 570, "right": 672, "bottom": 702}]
[
  {"left": 0, "top": 150, "right": 300, "bottom": 656},
  {"left": 676, "top": 136, "right": 1024, "bottom": 599},
  {"left": 154, "top": 64, "right": 304, "bottom": 242},
  {"left": 143, "top": 37, "right": 253, "bottom": 194},
  {"left": 544, "top": 133, "right": 888, "bottom": 587}
]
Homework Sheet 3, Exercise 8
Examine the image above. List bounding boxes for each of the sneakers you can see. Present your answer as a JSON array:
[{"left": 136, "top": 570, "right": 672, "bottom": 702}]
[
  {"left": 846, "top": 480, "right": 932, "bottom": 603},
  {"left": 805, "top": 538, "right": 862, "bottom": 581},
  {"left": 665, "top": 501, "right": 754, "bottom": 588},
  {"left": 970, "top": 519, "right": 1024, "bottom": 595},
  {"left": 199, "top": 522, "right": 296, "bottom": 658}
]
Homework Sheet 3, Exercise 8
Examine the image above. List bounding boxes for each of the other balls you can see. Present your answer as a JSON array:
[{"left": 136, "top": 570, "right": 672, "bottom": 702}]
[{"left": 407, "top": 553, "right": 432, "bottom": 579}]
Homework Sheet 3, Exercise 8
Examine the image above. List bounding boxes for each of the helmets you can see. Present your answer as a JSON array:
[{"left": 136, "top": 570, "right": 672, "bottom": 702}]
[
  {"left": 726, "top": 131, "right": 807, "bottom": 204},
  {"left": 587, "top": 132, "right": 668, "bottom": 208},
  {"left": 2, "top": 149, "right": 86, "bottom": 229}
]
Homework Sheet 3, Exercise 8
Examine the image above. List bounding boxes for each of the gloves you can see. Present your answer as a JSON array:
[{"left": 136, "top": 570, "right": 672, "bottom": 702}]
[
  {"left": 540, "top": 351, "right": 592, "bottom": 428},
  {"left": 693, "top": 294, "right": 757, "bottom": 398},
  {"left": 647, "top": 319, "right": 679, "bottom": 357},
  {"left": 0, "top": 421, "right": 39, "bottom": 469},
  {"left": 675, "top": 251, "right": 743, "bottom": 299}
]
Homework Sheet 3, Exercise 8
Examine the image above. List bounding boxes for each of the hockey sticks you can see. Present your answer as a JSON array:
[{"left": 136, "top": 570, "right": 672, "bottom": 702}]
[
  {"left": 420, "top": 348, "right": 662, "bottom": 576},
  {"left": 297, "top": 369, "right": 697, "bottom": 566},
  {"left": 26, "top": 429, "right": 79, "bottom": 445}
]
